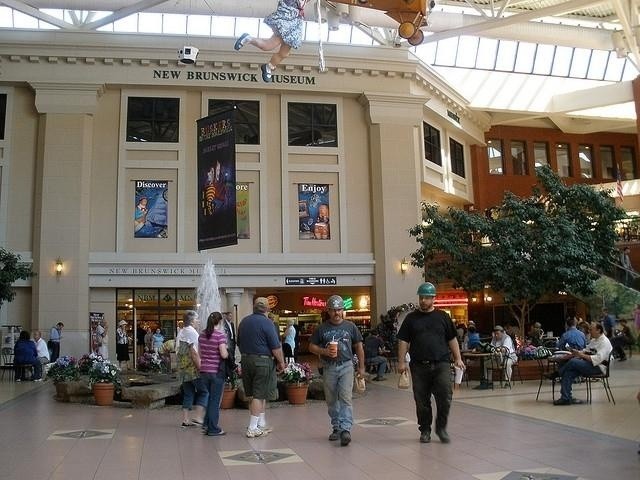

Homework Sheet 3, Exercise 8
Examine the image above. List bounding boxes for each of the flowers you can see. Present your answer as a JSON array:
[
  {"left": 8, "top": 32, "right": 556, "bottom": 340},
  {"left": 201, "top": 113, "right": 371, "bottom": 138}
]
[
  {"left": 224, "top": 368, "right": 243, "bottom": 389},
  {"left": 279, "top": 361, "right": 314, "bottom": 386},
  {"left": 46, "top": 352, "right": 165, "bottom": 384}
]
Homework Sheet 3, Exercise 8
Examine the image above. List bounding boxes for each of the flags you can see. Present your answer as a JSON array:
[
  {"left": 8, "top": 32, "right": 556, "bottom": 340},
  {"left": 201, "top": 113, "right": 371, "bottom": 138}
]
[{"left": 616, "top": 168, "right": 624, "bottom": 202}]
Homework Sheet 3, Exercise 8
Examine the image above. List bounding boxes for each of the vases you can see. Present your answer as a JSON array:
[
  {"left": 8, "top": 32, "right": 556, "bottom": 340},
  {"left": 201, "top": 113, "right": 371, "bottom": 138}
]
[
  {"left": 93, "top": 383, "right": 114, "bottom": 406},
  {"left": 219, "top": 387, "right": 237, "bottom": 409},
  {"left": 285, "top": 382, "right": 308, "bottom": 404}
]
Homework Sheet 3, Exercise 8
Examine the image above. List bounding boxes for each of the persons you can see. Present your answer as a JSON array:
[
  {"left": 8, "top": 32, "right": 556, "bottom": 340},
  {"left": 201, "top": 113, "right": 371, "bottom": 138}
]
[
  {"left": 480, "top": 325, "right": 518, "bottom": 389},
  {"left": 235, "top": 1, "right": 307, "bottom": 82},
  {"left": 97, "top": 331, "right": 108, "bottom": 360},
  {"left": 32, "top": 331, "right": 50, "bottom": 380},
  {"left": 631, "top": 303, "right": 640, "bottom": 333},
  {"left": 394, "top": 283, "right": 468, "bottom": 444},
  {"left": 143, "top": 327, "right": 153, "bottom": 347},
  {"left": 452, "top": 306, "right": 636, "bottom": 363},
  {"left": 197, "top": 311, "right": 229, "bottom": 436},
  {"left": 49, "top": 322, "right": 65, "bottom": 362},
  {"left": 13, "top": 331, "right": 42, "bottom": 382},
  {"left": 237, "top": 297, "right": 287, "bottom": 438},
  {"left": 116, "top": 320, "right": 131, "bottom": 370},
  {"left": 224, "top": 312, "right": 236, "bottom": 363},
  {"left": 222, "top": 313, "right": 227, "bottom": 321},
  {"left": 134, "top": 198, "right": 147, "bottom": 233},
  {"left": 151, "top": 328, "right": 164, "bottom": 354},
  {"left": 175, "top": 309, "right": 209, "bottom": 427},
  {"left": 620, "top": 246, "right": 633, "bottom": 286},
  {"left": 308, "top": 294, "right": 365, "bottom": 446},
  {"left": 310, "top": 203, "right": 330, "bottom": 239},
  {"left": 282, "top": 319, "right": 296, "bottom": 356},
  {"left": 544, "top": 322, "right": 613, "bottom": 405},
  {"left": 288, "top": 319, "right": 299, "bottom": 363},
  {"left": 364, "top": 330, "right": 387, "bottom": 381}
]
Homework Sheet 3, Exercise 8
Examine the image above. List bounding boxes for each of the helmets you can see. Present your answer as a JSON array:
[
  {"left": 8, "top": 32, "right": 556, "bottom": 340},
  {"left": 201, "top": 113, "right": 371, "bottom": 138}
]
[
  {"left": 325, "top": 294, "right": 345, "bottom": 310},
  {"left": 416, "top": 281, "right": 437, "bottom": 297}
]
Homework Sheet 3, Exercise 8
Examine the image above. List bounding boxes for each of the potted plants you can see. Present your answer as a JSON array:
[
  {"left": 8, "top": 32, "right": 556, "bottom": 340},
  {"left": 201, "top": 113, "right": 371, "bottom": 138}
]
[{"left": 53, "top": 381, "right": 88, "bottom": 404}]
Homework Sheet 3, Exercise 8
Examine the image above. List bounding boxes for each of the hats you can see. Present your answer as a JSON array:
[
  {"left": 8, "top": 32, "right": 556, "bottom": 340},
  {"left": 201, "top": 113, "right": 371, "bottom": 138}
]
[
  {"left": 494, "top": 325, "right": 504, "bottom": 331},
  {"left": 254, "top": 296, "right": 269, "bottom": 308},
  {"left": 118, "top": 319, "right": 129, "bottom": 326}
]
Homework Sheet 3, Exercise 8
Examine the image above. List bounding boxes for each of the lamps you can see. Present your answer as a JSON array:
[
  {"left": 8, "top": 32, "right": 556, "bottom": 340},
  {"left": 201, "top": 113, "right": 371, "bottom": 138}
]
[
  {"left": 399, "top": 256, "right": 409, "bottom": 273},
  {"left": 55, "top": 255, "right": 63, "bottom": 275}
]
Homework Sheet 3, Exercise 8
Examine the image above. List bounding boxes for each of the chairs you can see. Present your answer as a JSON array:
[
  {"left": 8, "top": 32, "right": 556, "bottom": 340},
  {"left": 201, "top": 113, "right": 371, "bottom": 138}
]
[
  {"left": 455, "top": 324, "right": 632, "bottom": 407},
  {"left": 0, "top": 347, "right": 44, "bottom": 383}
]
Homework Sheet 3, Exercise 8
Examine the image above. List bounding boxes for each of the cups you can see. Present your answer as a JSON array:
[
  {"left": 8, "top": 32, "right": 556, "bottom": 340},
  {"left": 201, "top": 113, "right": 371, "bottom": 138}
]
[
  {"left": 329, "top": 342, "right": 339, "bottom": 358},
  {"left": 454, "top": 366, "right": 463, "bottom": 385}
]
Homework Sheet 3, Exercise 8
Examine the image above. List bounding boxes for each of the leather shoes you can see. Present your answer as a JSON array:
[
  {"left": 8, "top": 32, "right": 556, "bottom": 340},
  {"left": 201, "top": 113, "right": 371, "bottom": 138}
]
[
  {"left": 435, "top": 427, "right": 451, "bottom": 444},
  {"left": 553, "top": 398, "right": 572, "bottom": 406},
  {"left": 543, "top": 372, "right": 555, "bottom": 381},
  {"left": 328, "top": 429, "right": 352, "bottom": 447},
  {"left": 420, "top": 429, "right": 431, "bottom": 443}
]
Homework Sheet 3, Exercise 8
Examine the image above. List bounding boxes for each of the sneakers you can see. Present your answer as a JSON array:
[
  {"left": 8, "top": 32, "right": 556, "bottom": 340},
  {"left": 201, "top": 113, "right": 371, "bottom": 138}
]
[
  {"left": 199, "top": 426, "right": 227, "bottom": 437},
  {"left": 244, "top": 426, "right": 274, "bottom": 438},
  {"left": 180, "top": 421, "right": 192, "bottom": 428},
  {"left": 190, "top": 416, "right": 204, "bottom": 427},
  {"left": 33, "top": 378, "right": 43, "bottom": 382},
  {"left": 15, "top": 378, "right": 21, "bottom": 382},
  {"left": 504, "top": 382, "right": 510, "bottom": 389}
]
